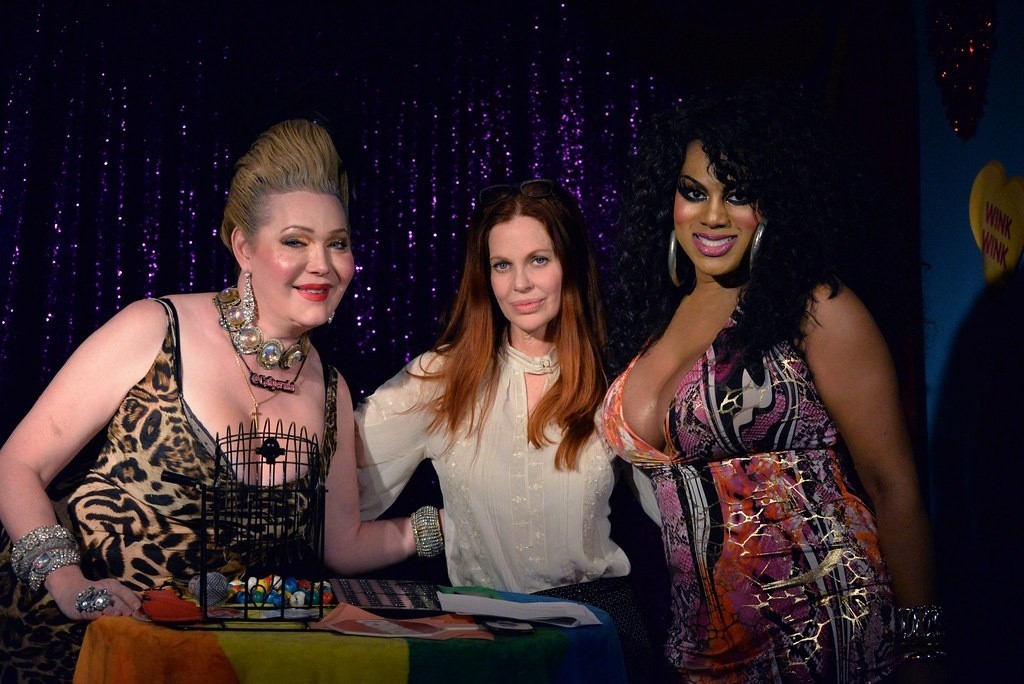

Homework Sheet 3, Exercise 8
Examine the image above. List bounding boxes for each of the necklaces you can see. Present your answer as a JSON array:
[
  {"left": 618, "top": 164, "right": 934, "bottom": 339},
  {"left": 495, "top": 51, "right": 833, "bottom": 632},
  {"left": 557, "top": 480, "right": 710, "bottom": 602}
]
[
  {"left": 213, "top": 296, "right": 312, "bottom": 394},
  {"left": 225, "top": 328, "right": 279, "bottom": 429},
  {"left": 219, "top": 286, "right": 312, "bottom": 368}
]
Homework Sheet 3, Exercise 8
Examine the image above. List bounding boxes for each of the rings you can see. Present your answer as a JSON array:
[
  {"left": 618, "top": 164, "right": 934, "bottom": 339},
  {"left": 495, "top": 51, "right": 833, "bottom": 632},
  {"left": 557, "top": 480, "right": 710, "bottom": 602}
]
[
  {"left": 77, "top": 585, "right": 112, "bottom": 613},
  {"left": 0, "top": 122, "right": 445, "bottom": 684}
]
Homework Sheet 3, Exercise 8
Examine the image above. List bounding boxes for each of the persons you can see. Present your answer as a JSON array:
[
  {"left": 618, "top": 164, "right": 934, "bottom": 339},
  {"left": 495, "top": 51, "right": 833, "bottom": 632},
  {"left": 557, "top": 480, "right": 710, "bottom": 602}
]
[
  {"left": 601, "top": 113, "right": 951, "bottom": 684},
  {"left": 353, "top": 179, "right": 666, "bottom": 622}
]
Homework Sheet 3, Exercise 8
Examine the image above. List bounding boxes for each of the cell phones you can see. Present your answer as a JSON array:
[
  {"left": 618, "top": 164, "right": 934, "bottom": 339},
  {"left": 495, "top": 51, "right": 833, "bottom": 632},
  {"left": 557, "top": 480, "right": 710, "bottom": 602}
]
[{"left": 139, "top": 588, "right": 201, "bottom": 624}]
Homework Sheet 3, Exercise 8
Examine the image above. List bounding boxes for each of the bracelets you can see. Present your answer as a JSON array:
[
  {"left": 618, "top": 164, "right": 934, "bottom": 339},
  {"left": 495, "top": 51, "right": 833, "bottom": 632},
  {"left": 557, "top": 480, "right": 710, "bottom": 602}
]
[
  {"left": 10, "top": 525, "right": 81, "bottom": 592},
  {"left": 889, "top": 601, "right": 948, "bottom": 666},
  {"left": 411, "top": 505, "right": 444, "bottom": 559}
]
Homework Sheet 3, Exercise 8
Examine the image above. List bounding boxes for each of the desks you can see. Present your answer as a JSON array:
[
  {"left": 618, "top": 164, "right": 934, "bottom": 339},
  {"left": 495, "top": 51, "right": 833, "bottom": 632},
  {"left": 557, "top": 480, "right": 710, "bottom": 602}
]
[{"left": 84, "top": 576, "right": 624, "bottom": 684}]
